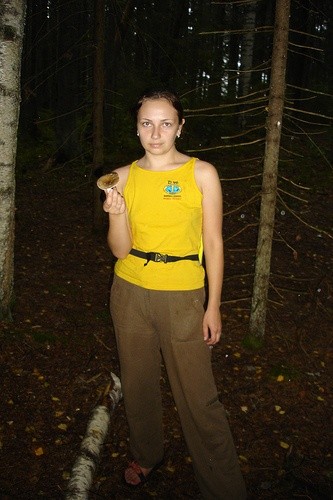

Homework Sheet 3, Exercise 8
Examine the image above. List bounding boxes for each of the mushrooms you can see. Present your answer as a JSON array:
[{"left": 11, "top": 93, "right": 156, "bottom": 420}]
[{"left": 97, "top": 173, "right": 119, "bottom": 194}]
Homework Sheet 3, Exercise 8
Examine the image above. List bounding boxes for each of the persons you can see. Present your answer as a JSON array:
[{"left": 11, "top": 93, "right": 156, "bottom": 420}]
[{"left": 103, "top": 89, "right": 246, "bottom": 500}]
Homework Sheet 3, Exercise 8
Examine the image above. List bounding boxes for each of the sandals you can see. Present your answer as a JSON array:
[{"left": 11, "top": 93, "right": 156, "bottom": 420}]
[{"left": 122, "top": 455, "right": 167, "bottom": 488}]
